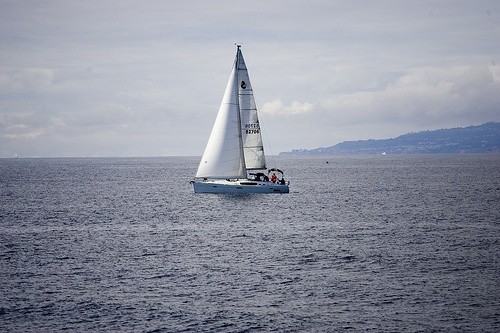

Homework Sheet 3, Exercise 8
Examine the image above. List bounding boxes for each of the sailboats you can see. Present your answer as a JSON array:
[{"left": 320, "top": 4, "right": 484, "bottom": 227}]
[{"left": 190, "top": 43, "right": 293, "bottom": 196}]
